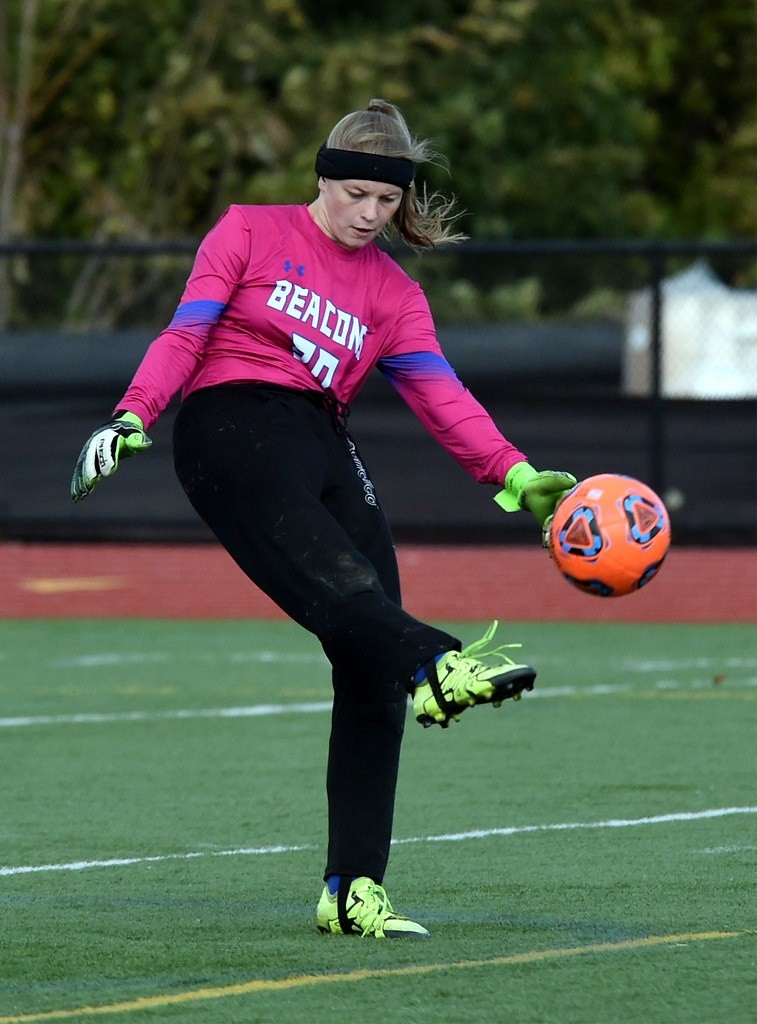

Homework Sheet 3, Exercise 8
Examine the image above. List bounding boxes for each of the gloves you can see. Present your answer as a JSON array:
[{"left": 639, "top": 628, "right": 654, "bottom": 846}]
[
  {"left": 70, "top": 411, "right": 151, "bottom": 505},
  {"left": 494, "top": 462, "right": 576, "bottom": 529}
]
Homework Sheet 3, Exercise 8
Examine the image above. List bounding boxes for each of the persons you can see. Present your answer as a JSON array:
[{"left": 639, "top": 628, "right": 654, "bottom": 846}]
[{"left": 64, "top": 97, "right": 584, "bottom": 941}]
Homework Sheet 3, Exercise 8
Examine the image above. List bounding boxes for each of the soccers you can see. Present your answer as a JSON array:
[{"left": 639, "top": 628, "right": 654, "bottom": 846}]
[{"left": 550, "top": 473, "right": 672, "bottom": 598}]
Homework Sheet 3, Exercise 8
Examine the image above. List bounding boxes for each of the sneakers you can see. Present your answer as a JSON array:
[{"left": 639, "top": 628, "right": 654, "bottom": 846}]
[
  {"left": 314, "top": 876, "right": 430, "bottom": 938},
  {"left": 412, "top": 648, "right": 536, "bottom": 729}
]
